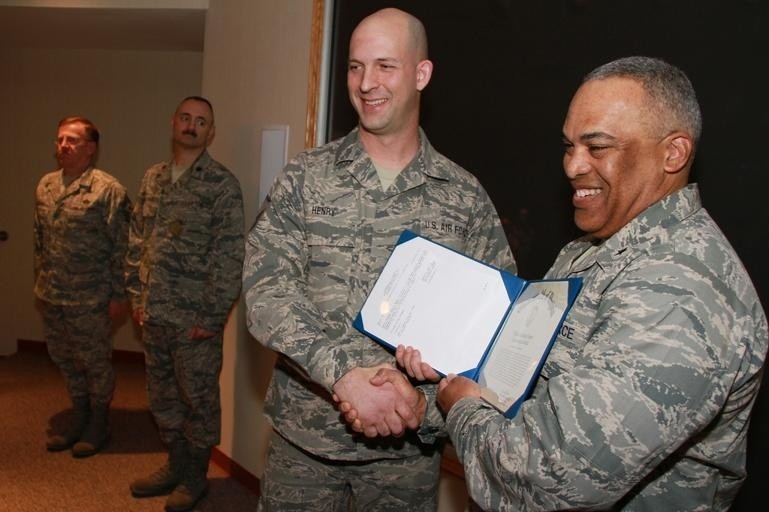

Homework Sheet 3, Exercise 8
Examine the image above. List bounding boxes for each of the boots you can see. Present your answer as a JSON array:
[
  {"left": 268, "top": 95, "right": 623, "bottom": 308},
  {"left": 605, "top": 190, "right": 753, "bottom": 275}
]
[
  {"left": 45, "top": 410, "right": 89, "bottom": 454},
  {"left": 72, "top": 410, "right": 115, "bottom": 459},
  {"left": 163, "top": 445, "right": 210, "bottom": 512},
  {"left": 129, "top": 443, "right": 186, "bottom": 498}
]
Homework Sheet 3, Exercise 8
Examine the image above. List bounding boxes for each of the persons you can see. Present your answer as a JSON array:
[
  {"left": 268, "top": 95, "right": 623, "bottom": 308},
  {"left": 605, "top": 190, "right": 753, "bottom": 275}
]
[
  {"left": 329, "top": 55, "right": 769, "bottom": 512},
  {"left": 239, "top": 6, "right": 520, "bottom": 511},
  {"left": 128, "top": 93, "right": 244, "bottom": 511},
  {"left": 30, "top": 114, "right": 133, "bottom": 459}
]
